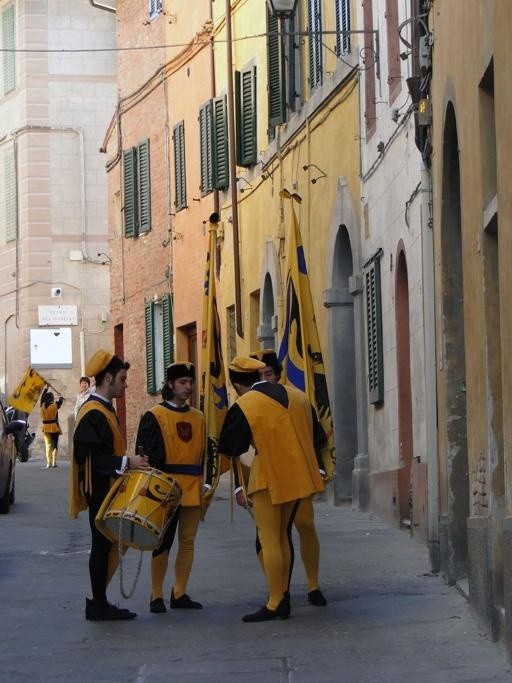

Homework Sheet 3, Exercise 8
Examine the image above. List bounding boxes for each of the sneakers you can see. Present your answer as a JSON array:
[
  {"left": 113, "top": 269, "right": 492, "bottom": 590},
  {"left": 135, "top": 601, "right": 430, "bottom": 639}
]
[
  {"left": 46, "top": 464, "right": 58, "bottom": 468},
  {"left": 86, "top": 597, "right": 129, "bottom": 619},
  {"left": 170, "top": 588, "right": 202, "bottom": 609},
  {"left": 308, "top": 590, "right": 327, "bottom": 606},
  {"left": 243, "top": 591, "right": 290, "bottom": 622},
  {"left": 150, "top": 593, "right": 167, "bottom": 613}
]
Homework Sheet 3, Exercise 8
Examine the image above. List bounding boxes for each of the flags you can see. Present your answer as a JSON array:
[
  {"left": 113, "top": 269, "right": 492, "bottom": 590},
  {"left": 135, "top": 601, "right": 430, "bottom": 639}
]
[
  {"left": 7, "top": 365, "right": 47, "bottom": 416},
  {"left": 198, "top": 224, "right": 231, "bottom": 522},
  {"left": 278, "top": 200, "right": 336, "bottom": 483}
]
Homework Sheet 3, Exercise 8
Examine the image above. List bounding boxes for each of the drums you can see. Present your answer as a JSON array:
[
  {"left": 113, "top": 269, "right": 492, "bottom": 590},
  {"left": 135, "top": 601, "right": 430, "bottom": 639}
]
[{"left": 103, "top": 466, "right": 182, "bottom": 551}]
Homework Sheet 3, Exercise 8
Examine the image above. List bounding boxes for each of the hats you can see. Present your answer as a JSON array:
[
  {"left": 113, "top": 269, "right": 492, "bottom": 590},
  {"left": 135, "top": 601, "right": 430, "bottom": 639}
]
[
  {"left": 229, "top": 356, "right": 266, "bottom": 381},
  {"left": 85, "top": 349, "right": 122, "bottom": 379},
  {"left": 249, "top": 349, "right": 278, "bottom": 364},
  {"left": 167, "top": 361, "right": 195, "bottom": 380},
  {"left": 44, "top": 392, "right": 54, "bottom": 402}
]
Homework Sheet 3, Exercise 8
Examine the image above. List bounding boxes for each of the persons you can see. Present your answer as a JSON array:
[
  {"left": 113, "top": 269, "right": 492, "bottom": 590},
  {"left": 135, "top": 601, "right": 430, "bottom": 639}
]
[
  {"left": 134, "top": 361, "right": 215, "bottom": 614},
  {"left": 40, "top": 384, "right": 63, "bottom": 469},
  {"left": 74, "top": 377, "right": 96, "bottom": 419},
  {"left": 69, "top": 350, "right": 151, "bottom": 622},
  {"left": 218, "top": 356, "right": 329, "bottom": 622},
  {"left": 228, "top": 348, "right": 327, "bottom": 607}
]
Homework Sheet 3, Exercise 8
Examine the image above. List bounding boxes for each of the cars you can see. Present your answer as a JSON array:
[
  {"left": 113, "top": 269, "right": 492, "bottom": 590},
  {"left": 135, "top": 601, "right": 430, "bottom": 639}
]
[{"left": 0, "top": 401, "right": 26, "bottom": 515}]
